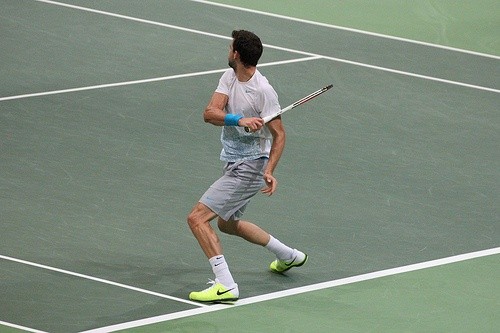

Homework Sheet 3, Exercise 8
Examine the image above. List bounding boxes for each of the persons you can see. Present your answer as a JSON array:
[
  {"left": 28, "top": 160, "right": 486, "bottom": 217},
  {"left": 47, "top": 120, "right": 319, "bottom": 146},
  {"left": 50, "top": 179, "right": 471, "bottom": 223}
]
[{"left": 187, "top": 28, "right": 308, "bottom": 303}]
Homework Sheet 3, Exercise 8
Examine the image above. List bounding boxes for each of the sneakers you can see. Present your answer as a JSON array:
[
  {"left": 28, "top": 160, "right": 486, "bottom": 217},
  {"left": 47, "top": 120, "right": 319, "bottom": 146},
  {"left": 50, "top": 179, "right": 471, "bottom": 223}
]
[
  {"left": 189, "top": 278, "right": 239, "bottom": 302},
  {"left": 269, "top": 249, "right": 308, "bottom": 273}
]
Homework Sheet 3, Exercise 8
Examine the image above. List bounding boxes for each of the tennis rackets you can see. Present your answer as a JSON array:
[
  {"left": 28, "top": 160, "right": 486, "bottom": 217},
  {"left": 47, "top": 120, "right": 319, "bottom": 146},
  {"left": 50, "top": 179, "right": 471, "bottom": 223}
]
[{"left": 244, "top": 84, "right": 333, "bottom": 133}]
